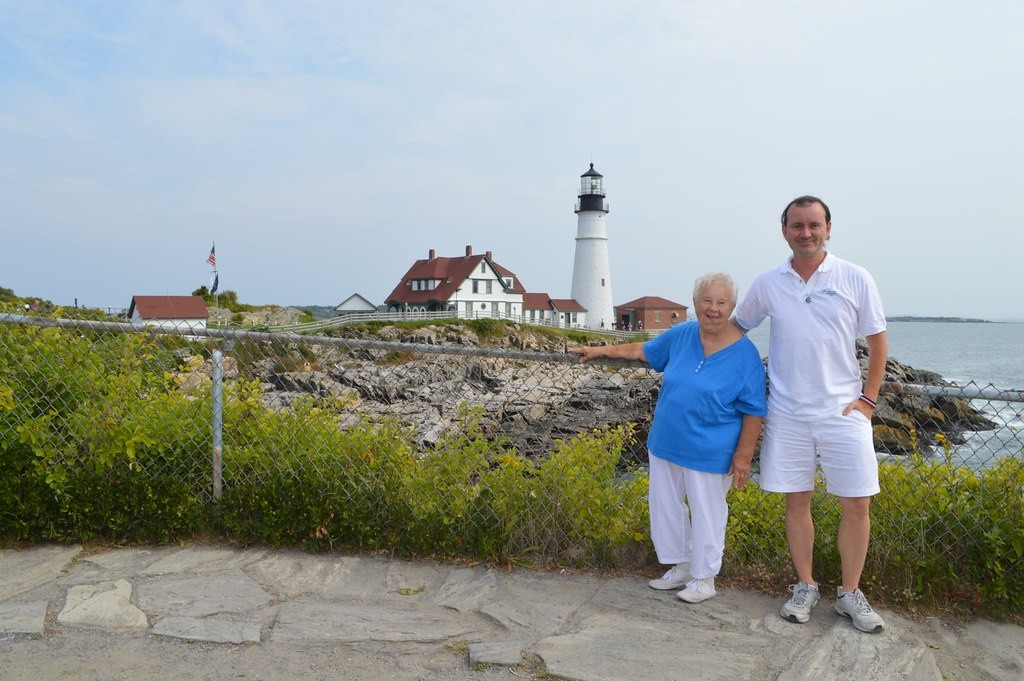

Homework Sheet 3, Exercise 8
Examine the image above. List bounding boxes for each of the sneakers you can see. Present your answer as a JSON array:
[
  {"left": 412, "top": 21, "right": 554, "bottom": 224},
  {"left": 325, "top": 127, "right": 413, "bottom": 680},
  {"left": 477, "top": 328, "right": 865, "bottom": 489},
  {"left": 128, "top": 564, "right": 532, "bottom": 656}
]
[
  {"left": 780, "top": 582, "right": 820, "bottom": 623},
  {"left": 833, "top": 586, "right": 886, "bottom": 634},
  {"left": 648, "top": 566, "right": 694, "bottom": 590},
  {"left": 676, "top": 579, "right": 716, "bottom": 604}
]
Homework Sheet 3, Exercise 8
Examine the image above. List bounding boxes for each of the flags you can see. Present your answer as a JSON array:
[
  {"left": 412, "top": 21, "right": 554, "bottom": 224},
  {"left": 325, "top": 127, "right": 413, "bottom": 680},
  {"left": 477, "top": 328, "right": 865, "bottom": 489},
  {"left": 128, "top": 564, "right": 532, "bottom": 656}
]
[
  {"left": 211, "top": 273, "right": 218, "bottom": 293},
  {"left": 206, "top": 246, "right": 216, "bottom": 266}
]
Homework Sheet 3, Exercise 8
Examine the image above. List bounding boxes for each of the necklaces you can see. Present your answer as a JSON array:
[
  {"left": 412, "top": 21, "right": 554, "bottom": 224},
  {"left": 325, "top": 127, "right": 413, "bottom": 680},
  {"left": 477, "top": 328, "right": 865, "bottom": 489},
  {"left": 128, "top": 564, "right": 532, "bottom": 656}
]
[{"left": 792, "top": 261, "right": 824, "bottom": 304}]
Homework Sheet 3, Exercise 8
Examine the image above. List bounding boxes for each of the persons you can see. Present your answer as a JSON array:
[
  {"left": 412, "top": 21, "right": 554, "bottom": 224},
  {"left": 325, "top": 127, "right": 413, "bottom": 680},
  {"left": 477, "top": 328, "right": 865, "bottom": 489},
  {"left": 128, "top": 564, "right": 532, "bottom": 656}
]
[
  {"left": 621, "top": 318, "right": 643, "bottom": 331},
  {"left": 728, "top": 196, "right": 888, "bottom": 633},
  {"left": 601, "top": 318, "right": 605, "bottom": 329},
  {"left": 567, "top": 272, "right": 767, "bottom": 604}
]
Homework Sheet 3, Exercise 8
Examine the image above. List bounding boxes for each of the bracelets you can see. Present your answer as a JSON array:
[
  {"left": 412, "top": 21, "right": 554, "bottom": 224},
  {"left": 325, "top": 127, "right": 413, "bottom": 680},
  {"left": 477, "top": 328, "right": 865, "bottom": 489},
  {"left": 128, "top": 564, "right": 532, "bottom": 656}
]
[{"left": 858, "top": 395, "right": 876, "bottom": 409}]
